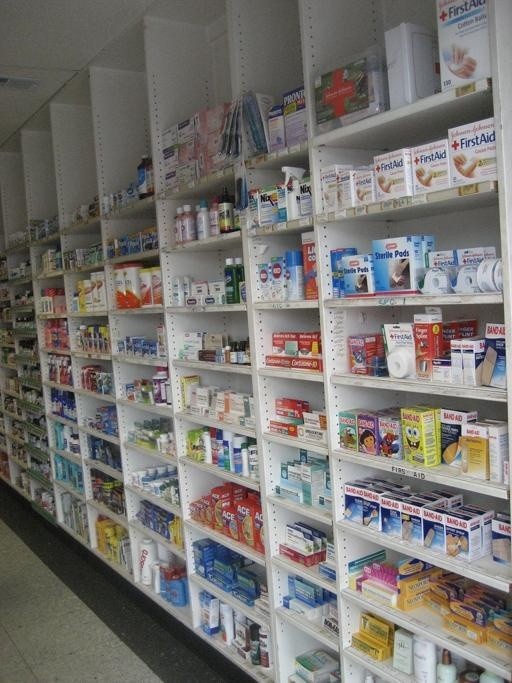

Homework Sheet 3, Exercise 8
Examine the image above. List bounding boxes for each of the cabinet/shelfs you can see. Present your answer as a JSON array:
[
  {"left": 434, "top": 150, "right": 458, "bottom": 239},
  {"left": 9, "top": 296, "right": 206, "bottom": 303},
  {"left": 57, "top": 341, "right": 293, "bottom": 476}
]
[{"left": 0, "top": 2, "right": 512, "bottom": 681}]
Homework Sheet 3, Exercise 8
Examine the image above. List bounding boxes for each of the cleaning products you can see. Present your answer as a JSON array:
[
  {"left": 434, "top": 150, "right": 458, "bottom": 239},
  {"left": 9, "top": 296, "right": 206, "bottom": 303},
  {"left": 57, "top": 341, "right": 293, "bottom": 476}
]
[{"left": 281, "top": 164, "right": 307, "bottom": 221}]
